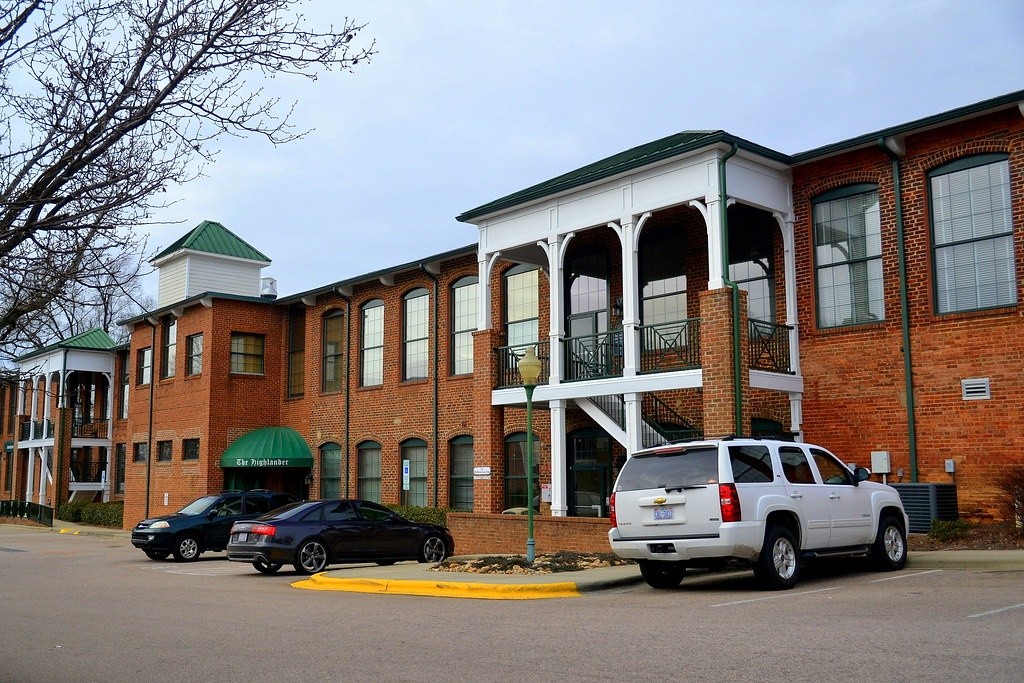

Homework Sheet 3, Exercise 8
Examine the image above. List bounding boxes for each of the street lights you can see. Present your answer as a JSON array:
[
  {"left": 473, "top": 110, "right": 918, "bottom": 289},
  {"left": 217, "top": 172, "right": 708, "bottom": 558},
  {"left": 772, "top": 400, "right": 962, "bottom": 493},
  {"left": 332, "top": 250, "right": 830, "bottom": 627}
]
[{"left": 518, "top": 345, "right": 543, "bottom": 562}]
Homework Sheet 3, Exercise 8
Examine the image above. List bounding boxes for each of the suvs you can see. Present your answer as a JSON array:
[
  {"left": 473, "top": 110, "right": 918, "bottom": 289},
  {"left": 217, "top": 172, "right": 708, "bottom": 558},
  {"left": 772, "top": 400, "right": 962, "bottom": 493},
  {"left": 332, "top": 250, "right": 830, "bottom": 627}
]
[
  {"left": 607, "top": 434, "right": 910, "bottom": 592},
  {"left": 130, "top": 488, "right": 299, "bottom": 562}
]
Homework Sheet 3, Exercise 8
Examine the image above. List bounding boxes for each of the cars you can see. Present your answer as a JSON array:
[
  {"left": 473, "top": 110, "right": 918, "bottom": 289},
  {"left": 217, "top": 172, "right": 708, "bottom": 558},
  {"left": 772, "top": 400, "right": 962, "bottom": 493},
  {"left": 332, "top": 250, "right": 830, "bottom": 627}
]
[{"left": 227, "top": 498, "right": 455, "bottom": 576}]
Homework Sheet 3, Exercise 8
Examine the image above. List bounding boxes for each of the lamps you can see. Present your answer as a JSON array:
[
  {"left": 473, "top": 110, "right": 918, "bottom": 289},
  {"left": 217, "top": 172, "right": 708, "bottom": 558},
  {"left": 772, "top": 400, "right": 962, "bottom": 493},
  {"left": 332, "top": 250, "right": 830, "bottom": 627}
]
[{"left": 612, "top": 297, "right": 621, "bottom": 316}]
